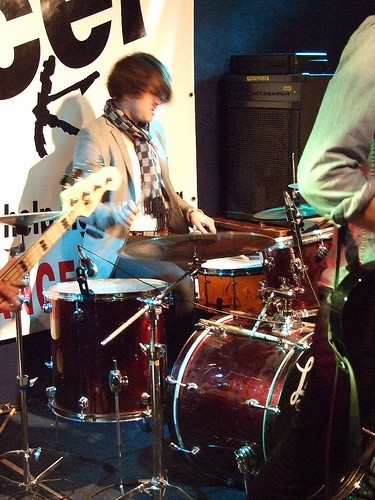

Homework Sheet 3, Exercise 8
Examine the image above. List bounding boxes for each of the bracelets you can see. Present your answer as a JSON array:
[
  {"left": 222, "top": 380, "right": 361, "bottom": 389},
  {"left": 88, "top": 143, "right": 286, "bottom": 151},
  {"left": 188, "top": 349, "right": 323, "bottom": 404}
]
[{"left": 185, "top": 208, "right": 204, "bottom": 223}]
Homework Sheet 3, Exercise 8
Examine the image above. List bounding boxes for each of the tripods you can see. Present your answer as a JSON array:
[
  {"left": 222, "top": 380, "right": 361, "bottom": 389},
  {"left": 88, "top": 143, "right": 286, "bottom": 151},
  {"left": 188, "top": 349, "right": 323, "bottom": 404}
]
[{"left": 0, "top": 229, "right": 201, "bottom": 500}]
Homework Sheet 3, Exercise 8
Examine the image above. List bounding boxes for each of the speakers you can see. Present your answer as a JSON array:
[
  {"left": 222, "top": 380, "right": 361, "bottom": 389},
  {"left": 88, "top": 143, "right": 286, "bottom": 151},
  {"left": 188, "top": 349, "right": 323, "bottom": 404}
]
[{"left": 221, "top": 106, "right": 299, "bottom": 226}]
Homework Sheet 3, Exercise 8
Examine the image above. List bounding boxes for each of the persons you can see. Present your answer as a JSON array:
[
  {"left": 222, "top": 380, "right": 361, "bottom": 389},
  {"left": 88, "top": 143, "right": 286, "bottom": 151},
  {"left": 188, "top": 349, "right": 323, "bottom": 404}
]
[
  {"left": 296, "top": 15, "right": 375, "bottom": 232},
  {"left": 73, "top": 53, "right": 217, "bottom": 370}
]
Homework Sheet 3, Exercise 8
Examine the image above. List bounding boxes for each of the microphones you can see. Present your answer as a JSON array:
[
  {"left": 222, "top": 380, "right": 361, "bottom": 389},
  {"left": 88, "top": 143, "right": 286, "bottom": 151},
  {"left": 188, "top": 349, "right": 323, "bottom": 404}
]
[
  {"left": 283, "top": 192, "right": 301, "bottom": 223},
  {"left": 77, "top": 247, "right": 98, "bottom": 277}
]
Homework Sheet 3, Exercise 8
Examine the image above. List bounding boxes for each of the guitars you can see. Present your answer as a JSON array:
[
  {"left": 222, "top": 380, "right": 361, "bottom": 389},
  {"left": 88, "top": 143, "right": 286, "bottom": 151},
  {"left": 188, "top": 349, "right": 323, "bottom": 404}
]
[{"left": 0, "top": 158, "right": 122, "bottom": 304}]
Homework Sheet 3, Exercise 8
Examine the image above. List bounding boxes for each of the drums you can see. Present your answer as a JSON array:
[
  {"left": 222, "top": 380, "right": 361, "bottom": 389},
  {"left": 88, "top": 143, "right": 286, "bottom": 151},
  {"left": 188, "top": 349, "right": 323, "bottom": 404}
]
[
  {"left": 196, "top": 255, "right": 272, "bottom": 315},
  {"left": 269, "top": 228, "right": 348, "bottom": 318},
  {"left": 264, "top": 216, "right": 338, "bottom": 237},
  {"left": 47, "top": 278, "right": 169, "bottom": 423},
  {"left": 167, "top": 313, "right": 375, "bottom": 500}
]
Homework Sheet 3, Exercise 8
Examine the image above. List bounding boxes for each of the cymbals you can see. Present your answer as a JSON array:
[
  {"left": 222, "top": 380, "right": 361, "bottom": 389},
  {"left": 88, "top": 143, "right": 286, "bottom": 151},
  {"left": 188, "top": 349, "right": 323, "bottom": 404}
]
[
  {"left": 253, "top": 206, "right": 326, "bottom": 223},
  {"left": 116, "top": 232, "right": 277, "bottom": 264},
  {"left": 0, "top": 211, "right": 64, "bottom": 226}
]
